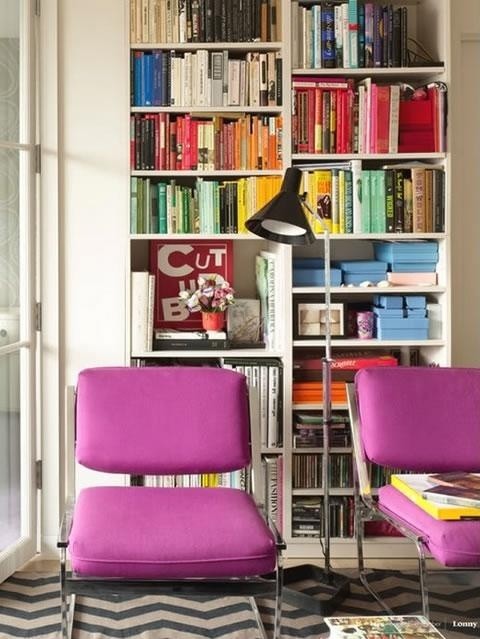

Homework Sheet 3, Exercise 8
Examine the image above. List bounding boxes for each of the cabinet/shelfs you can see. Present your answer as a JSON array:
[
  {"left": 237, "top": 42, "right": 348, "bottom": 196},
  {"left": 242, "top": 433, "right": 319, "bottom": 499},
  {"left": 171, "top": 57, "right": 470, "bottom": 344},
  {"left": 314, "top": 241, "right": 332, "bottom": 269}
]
[{"left": 128, "top": 2, "right": 453, "bottom": 558}]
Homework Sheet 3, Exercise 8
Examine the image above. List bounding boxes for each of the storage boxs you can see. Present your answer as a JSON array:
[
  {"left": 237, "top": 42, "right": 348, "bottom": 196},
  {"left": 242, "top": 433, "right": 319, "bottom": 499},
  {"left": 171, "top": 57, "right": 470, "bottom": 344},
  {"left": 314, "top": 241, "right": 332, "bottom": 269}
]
[
  {"left": 376, "top": 317, "right": 429, "bottom": 340},
  {"left": 339, "top": 259, "right": 388, "bottom": 284},
  {"left": 293, "top": 256, "right": 342, "bottom": 286}
]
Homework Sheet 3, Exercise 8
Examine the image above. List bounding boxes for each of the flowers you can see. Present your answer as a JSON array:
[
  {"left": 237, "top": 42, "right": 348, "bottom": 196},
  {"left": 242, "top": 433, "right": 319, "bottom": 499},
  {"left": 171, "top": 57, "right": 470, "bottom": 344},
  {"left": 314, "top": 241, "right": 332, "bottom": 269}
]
[{"left": 177, "top": 275, "right": 237, "bottom": 313}]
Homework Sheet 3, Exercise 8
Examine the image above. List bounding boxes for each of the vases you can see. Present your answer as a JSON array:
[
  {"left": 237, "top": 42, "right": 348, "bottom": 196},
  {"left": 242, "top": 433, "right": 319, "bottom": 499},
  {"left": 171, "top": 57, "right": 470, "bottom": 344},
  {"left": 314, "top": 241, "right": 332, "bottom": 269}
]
[{"left": 202, "top": 309, "right": 225, "bottom": 332}]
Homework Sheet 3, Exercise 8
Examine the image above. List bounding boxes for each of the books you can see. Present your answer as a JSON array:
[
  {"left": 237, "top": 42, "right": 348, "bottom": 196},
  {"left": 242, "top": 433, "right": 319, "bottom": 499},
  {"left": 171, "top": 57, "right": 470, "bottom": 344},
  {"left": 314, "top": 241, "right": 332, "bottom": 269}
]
[
  {"left": 292, "top": 453, "right": 424, "bottom": 538},
  {"left": 131, "top": 112, "right": 284, "bottom": 173},
  {"left": 298, "top": 237, "right": 440, "bottom": 340},
  {"left": 299, "top": 163, "right": 444, "bottom": 232},
  {"left": 142, "top": 458, "right": 280, "bottom": 540},
  {"left": 131, "top": 49, "right": 283, "bottom": 110},
  {"left": 292, "top": 0, "right": 410, "bottom": 69},
  {"left": 220, "top": 357, "right": 285, "bottom": 449},
  {"left": 290, "top": 76, "right": 448, "bottom": 155},
  {"left": 292, "top": 346, "right": 419, "bottom": 450},
  {"left": 255, "top": 248, "right": 276, "bottom": 348},
  {"left": 130, "top": 173, "right": 280, "bottom": 235},
  {"left": 130, "top": 0, "right": 280, "bottom": 46},
  {"left": 131, "top": 270, "right": 155, "bottom": 354},
  {"left": 391, "top": 471, "right": 480, "bottom": 521}
]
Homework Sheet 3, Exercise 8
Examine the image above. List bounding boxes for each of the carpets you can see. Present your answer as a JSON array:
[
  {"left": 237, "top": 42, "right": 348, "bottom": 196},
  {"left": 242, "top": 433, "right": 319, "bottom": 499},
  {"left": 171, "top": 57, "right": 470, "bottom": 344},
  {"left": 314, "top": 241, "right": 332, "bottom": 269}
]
[{"left": 0, "top": 566, "right": 480, "bottom": 639}]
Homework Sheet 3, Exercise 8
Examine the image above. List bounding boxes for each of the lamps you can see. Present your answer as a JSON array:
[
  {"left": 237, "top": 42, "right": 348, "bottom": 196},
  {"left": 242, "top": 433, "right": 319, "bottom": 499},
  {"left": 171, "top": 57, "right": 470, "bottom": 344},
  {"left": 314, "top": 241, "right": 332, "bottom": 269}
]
[{"left": 243, "top": 167, "right": 351, "bottom": 616}]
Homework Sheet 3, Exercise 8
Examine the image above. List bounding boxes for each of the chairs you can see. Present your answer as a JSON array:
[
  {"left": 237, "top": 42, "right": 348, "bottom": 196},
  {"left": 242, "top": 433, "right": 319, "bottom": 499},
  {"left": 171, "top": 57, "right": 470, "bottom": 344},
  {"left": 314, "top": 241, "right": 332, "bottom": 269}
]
[
  {"left": 344, "top": 367, "right": 480, "bottom": 622},
  {"left": 56, "top": 367, "right": 288, "bottom": 639}
]
